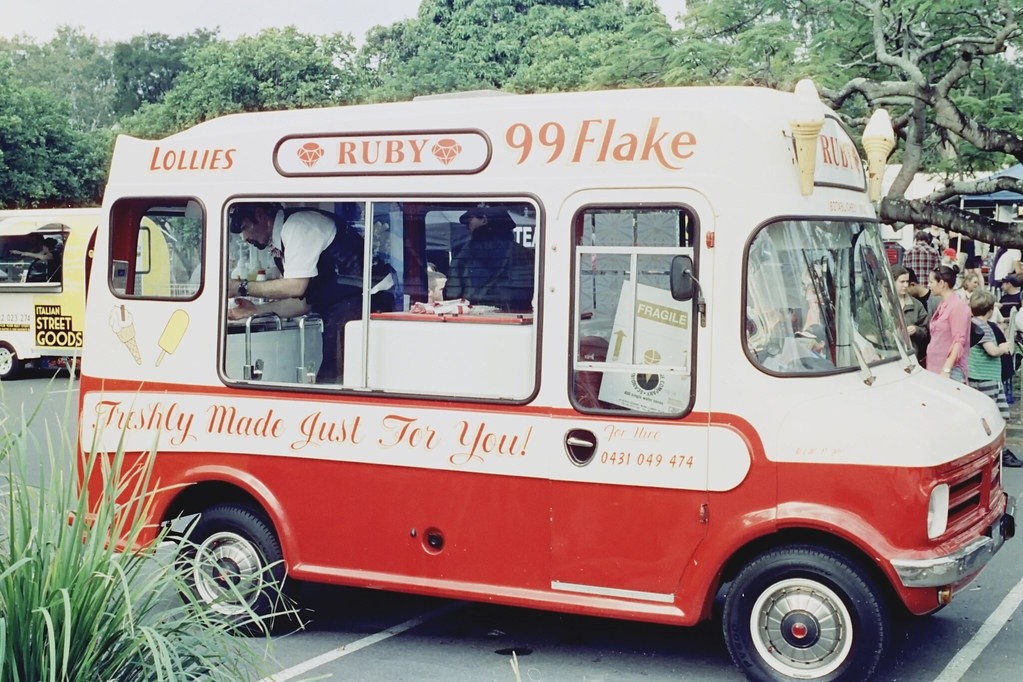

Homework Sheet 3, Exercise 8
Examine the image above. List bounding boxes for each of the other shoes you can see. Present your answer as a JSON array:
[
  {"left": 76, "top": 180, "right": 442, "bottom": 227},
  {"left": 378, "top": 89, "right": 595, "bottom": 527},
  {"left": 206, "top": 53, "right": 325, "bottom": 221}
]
[{"left": 1002, "top": 449, "right": 1023, "bottom": 468}]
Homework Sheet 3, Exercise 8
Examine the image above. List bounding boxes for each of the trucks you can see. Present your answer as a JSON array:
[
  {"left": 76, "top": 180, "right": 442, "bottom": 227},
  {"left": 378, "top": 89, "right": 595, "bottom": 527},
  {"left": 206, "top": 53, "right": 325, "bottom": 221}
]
[
  {"left": 67, "top": 79, "right": 1016, "bottom": 682},
  {"left": 0, "top": 208, "right": 172, "bottom": 382}
]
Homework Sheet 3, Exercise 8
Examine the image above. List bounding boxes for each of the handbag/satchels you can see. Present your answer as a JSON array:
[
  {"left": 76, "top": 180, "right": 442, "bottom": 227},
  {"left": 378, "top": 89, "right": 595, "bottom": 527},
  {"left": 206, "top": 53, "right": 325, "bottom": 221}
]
[{"left": 970, "top": 321, "right": 984, "bottom": 347}]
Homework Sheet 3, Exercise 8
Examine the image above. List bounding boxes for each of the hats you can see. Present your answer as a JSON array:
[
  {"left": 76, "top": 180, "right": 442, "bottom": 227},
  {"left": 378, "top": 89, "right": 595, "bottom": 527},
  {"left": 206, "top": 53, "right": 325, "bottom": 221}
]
[
  {"left": 1002, "top": 275, "right": 1018, "bottom": 288},
  {"left": 230, "top": 202, "right": 260, "bottom": 233},
  {"left": 458, "top": 204, "right": 516, "bottom": 228}
]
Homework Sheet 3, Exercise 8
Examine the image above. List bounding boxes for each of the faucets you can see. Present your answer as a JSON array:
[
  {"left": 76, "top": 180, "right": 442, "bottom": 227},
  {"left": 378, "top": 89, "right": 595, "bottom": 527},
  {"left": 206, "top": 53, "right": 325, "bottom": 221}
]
[
  {"left": 296, "top": 314, "right": 324, "bottom": 383},
  {"left": 243, "top": 312, "right": 282, "bottom": 380}
]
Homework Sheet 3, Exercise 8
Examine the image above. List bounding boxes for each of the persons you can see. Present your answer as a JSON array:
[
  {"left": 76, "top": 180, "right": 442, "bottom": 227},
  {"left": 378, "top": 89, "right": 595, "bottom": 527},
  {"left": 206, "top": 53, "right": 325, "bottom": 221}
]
[
  {"left": 228, "top": 203, "right": 396, "bottom": 384},
  {"left": 441, "top": 203, "right": 535, "bottom": 314},
  {"left": 9, "top": 237, "right": 56, "bottom": 260},
  {"left": 891, "top": 228, "right": 1023, "bottom": 466},
  {"left": 796, "top": 260, "right": 837, "bottom": 352}
]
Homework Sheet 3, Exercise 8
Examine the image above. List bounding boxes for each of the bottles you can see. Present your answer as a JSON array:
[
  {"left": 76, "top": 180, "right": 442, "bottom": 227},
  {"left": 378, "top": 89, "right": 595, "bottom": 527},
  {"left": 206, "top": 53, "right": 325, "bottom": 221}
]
[
  {"left": 232, "top": 260, "right": 249, "bottom": 280},
  {"left": 255, "top": 269, "right": 267, "bottom": 303}
]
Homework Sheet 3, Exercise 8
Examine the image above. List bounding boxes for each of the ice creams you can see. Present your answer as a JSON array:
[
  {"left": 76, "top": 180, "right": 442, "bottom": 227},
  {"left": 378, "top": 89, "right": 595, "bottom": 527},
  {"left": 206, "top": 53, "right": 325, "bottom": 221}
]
[
  {"left": 789, "top": 79, "right": 826, "bottom": 197},
  {"left": 861, "top": 109, "right": 896, "bottom": 200}
]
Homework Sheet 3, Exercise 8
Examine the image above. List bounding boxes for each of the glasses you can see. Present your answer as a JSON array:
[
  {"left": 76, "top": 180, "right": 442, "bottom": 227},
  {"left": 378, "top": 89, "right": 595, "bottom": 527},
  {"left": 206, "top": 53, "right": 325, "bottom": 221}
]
[{"left": 934, "top": 267, "right": 942, "bottom": 280}]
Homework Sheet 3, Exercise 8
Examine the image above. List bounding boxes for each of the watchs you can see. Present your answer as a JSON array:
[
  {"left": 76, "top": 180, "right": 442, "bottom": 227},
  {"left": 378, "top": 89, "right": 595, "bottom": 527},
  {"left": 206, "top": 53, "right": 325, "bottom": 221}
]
[{"left": 238, "top": 280, "right": 247, "bottom": 296}]
